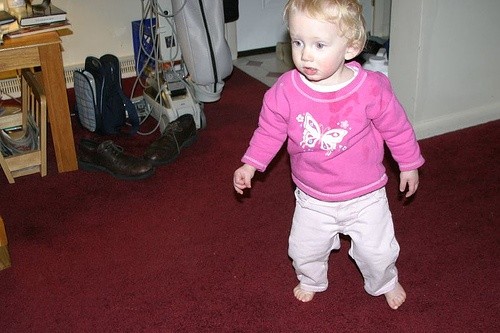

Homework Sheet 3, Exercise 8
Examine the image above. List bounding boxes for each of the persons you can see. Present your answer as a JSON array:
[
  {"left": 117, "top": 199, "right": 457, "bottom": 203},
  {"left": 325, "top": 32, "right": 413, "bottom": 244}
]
[{"left": 233, "top": 0, "right": 426, "bottom": 310}]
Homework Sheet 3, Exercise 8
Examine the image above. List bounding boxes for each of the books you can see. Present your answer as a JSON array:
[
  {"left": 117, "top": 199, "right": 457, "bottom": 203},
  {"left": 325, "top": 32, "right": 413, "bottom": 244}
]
[{"left": 0, "top": 3, "right": 72, "bottom": 43}]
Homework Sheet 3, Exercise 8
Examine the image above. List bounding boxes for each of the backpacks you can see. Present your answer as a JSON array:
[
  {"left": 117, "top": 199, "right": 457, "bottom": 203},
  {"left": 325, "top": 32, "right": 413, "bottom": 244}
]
[{"left": 73, "top": 54, "right": 138, "bottom": 137}]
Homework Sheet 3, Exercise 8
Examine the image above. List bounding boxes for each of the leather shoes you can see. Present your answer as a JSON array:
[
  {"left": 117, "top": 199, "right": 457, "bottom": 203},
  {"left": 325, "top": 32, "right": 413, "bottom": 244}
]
[
  {"left": 143, "top": 114, "right": 196, "bottom": 165},
  {"left": 76, "top": 139, "right": 153, "bottom": 179}
]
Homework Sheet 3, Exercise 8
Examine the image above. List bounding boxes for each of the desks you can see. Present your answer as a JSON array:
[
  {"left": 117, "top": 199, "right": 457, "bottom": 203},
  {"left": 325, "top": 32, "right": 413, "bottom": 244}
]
[{"left": 0, "top": 31, "right": 79, "bottom": 173}]
